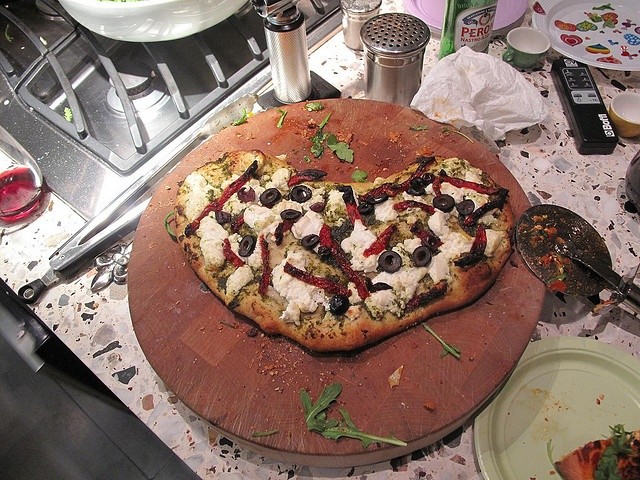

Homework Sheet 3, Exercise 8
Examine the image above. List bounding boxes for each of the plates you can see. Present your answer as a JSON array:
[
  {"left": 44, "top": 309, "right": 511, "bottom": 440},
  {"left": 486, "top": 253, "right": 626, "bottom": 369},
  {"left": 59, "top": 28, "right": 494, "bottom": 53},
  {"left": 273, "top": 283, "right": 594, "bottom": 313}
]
[
  {"left": 404, "top": 1, "right": 530, "bottom": 33},
  {"left": 474, "top": 337, "right": 636, "bottom": 479},
  {"left": 57, "top": 0, "right": 248, "bottom": 43},
  {"left": 531, "top": 0, "right": 640, "bottom": 71}
]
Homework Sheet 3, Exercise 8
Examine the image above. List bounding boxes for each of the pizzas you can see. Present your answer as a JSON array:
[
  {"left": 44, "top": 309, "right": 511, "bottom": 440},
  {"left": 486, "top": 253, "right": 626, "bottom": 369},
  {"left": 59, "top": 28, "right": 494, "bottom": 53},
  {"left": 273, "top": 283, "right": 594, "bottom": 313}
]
[
  {"left": 174, "top": 149, "right": 516, "bottom": 352},
  {"left": 555, "top": 429, "right": 639, "bottom": 480}
]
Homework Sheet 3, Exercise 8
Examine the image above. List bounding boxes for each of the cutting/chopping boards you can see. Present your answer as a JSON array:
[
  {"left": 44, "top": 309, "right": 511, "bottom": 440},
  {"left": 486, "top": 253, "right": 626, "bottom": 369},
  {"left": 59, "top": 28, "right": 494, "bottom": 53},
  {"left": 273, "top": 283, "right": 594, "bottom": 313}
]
[{"left": 129, "top": 97, "right": 547, "bottom": 465}]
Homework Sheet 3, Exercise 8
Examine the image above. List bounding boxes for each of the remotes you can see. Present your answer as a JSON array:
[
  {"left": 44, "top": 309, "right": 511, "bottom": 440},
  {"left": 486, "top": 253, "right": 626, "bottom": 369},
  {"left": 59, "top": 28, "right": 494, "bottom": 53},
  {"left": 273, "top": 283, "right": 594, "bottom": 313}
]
[{"left": 552, "top": 56, "right": 619, "bottom": 155}]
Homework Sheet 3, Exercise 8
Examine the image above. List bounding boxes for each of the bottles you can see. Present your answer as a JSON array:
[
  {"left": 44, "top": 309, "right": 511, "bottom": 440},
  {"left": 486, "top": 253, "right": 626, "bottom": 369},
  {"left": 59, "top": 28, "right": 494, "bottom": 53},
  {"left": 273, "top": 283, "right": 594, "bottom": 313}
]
[
  {"left": 340, "top": 1, "right": 381, "bottom": 52},
  {"left": 440, "top": 0, "right": 497, "bottom": 59},
  {"left": 358, "top": 11, "right": 430, "bottom": 106}
]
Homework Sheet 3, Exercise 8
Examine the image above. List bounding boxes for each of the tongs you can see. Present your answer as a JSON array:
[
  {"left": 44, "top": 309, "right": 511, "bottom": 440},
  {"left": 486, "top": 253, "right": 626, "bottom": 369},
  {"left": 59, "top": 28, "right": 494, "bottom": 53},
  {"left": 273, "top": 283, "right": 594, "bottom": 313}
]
[{"left": 19, "top": 94, "right": 276, "bottom": 306}]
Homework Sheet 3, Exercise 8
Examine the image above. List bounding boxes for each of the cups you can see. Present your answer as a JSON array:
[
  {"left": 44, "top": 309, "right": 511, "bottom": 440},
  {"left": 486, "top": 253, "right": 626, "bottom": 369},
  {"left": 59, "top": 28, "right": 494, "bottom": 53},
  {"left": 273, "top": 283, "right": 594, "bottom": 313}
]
[
  {"left": 610, "top": 92, "right": 640, "bottom": 138},
  {"left": 503, "top": 27, "right": 551, "bottom": 66},
  {"left": 1, "top": 126, "right": 44, "bottom": 223},
  {"left": 263, "top": 5, "right": 313, "bottom": 104}
]
